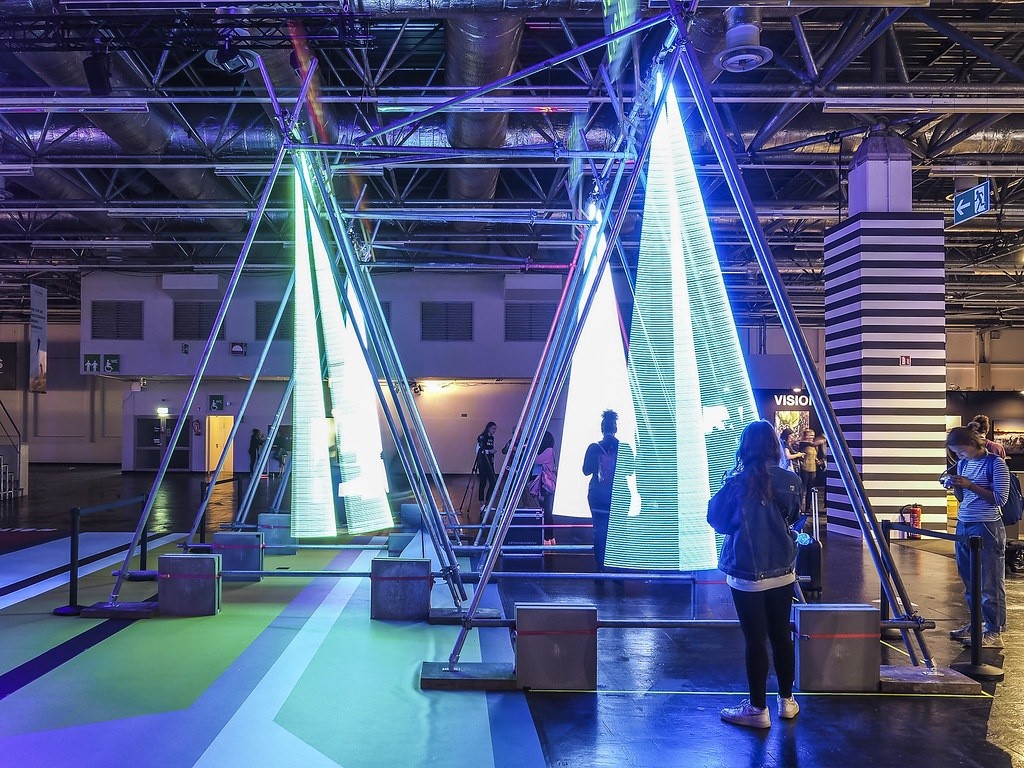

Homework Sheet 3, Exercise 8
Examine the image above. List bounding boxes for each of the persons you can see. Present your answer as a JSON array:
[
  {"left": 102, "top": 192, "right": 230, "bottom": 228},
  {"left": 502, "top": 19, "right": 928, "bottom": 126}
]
[
  {"left": 535, "top": 431, "right": 556, "bottom": 545},
  {"left": 706, "top": 417, "right": 799, "bottom": 729},
  {"left": 779, "top": 428, "right": 805, "bottom": 472},
  {"left": 501, "top": 426, "right": 533, "bottom": 508},
  {"left": 798, "top": 429, "right": 827, "bottom": 516},
  {"left": 475, "top": 421, "right": 498, "bottom": 512},
  {"left": 972, "top": 415, "right": 1005, "bottom": 460},
  {"left": 249, "top": 429, "right": 263, "bottom": 478},
  {"left": 944, "top": 422, "right": 1010, "bottom": 648},
  {"left": 273, "top": 429, "right": 286, "bottom": 477},
  {"left": 1007, "top": 434, "right": 1024, "bottom": 454},
  {"left": 582, "top": 410, "right": 634, "bottom": 586}
]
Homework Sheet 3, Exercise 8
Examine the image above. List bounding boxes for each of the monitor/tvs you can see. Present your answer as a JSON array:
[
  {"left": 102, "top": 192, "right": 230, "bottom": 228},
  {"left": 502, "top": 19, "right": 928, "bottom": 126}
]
[{"left": 268, "top": 424, "right": 292, "bottom": 451}]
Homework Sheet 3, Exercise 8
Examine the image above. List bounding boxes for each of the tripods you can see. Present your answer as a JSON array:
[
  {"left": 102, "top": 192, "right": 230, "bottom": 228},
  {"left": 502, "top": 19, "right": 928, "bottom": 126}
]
[{"left": 460, "top": 448, "right": 498, "bottom": 515}]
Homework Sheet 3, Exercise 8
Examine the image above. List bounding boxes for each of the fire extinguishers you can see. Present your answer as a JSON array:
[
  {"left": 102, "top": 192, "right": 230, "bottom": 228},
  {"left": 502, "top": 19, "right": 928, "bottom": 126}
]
[{"left": 901, "top": 503, "right": 924, "bottom": 540}]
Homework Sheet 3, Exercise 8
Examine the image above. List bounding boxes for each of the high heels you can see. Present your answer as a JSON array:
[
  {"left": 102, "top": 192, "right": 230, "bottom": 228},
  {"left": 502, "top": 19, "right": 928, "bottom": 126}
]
[{"left": 594, "top": 570, "right": 624, "bottom": 585}]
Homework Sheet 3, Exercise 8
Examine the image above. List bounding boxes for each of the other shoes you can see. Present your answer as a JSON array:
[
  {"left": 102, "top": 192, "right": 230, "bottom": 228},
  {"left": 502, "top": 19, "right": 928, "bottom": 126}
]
[
  {"left": 480, "top": 504, "right": 496, "bottom": 511},
  {"left": 544, "top": 538, "right": 556, "bottom": 545}
]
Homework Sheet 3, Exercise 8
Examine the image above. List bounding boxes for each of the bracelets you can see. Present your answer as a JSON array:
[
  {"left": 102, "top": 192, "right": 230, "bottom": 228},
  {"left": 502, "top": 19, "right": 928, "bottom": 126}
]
[{"left": 968, "top": 483, "right": 973, "bottom": 490}]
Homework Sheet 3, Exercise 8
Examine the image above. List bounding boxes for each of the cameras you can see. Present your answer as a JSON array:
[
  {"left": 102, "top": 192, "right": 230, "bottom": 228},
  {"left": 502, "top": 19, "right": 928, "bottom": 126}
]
[
  {"left": 478, "top": 434, "right": 487, "bottom": 448},
  {"left": 940, "top": 474, "right": 953, "bottom": 489}
]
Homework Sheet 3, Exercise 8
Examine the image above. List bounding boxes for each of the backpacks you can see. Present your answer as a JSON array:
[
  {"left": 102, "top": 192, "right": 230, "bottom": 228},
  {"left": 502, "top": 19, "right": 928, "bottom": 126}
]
[
  {"left": 595, "top": 442, "right": 618, "bottom": 484},
  {"left": 960, "top": 454, "right": 1024, "bottom": 525}
]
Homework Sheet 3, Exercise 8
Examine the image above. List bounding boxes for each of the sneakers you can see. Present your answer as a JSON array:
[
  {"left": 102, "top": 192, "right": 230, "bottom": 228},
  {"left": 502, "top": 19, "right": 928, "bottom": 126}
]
[
  {"left": 950, "top": 626, "right": 984, "bottom": 638},
  {"left": 963, "top": 633, "right": 1005, "bottom": 648},
  {"left": 777, "top": 694, "right": 800, "bottom": 718},
  {"left": 721, "top": 703, "right": 771, "bottom": 728}
]
